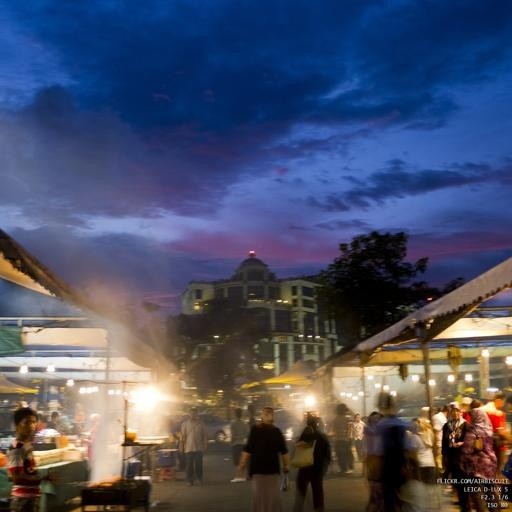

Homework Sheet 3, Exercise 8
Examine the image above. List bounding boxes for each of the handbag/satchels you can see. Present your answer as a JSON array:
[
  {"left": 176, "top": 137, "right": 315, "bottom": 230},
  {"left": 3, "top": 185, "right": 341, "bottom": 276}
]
[
  {"left": 291, "top": 440, "right": 316, "bottom": 467},
  {"left": 280, "top": 473, "right": 289, "bottom": 492},
  {"left": 467, "top": 425, "right": 484, "bottom": 451}
]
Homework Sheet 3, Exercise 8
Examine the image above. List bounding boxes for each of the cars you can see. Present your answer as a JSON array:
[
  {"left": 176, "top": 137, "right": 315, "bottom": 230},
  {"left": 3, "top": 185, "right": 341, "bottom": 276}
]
[
  {"left": 240, "top": 406, "right": 301, "bottom": 437},
  {"left": 195, "top": 412, "right": 235, "bottom": 444}
]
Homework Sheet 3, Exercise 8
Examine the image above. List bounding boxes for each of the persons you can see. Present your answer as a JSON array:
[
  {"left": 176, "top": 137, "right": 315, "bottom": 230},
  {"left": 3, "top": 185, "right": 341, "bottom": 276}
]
[{"left": 7, "top": 389, "right": 511, "bottom": 512}]
